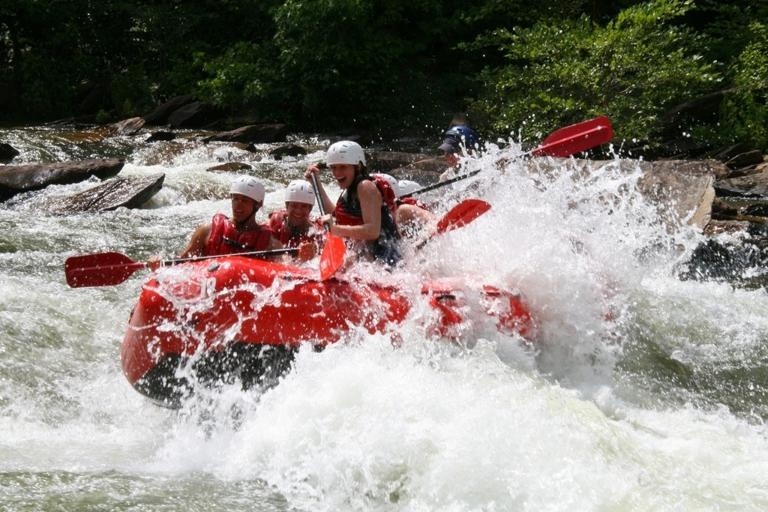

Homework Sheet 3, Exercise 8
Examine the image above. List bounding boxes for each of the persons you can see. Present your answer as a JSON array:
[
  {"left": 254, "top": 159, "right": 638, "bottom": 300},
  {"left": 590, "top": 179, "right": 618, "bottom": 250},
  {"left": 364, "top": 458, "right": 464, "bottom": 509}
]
[
  {"left": 147, "top": 174, "right": 317, "bottom": 273},
  {"left": 267, "top": 180, "right": 321, "bottom": 267},
  {"left": 437, "top": 125, "right": 478, "bottom": 196},
  {"left": 370, "top": 172, "right": 435, "bottom": 233},
  {"left": 303, "top": 140, "right": 400, "bottom": 270}
]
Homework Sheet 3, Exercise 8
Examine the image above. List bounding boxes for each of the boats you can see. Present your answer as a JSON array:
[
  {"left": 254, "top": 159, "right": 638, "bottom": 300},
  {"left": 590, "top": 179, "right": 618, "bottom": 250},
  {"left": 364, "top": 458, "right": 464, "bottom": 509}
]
[{"left": 120, "top": 191, "right": 556, "bottom": 415}]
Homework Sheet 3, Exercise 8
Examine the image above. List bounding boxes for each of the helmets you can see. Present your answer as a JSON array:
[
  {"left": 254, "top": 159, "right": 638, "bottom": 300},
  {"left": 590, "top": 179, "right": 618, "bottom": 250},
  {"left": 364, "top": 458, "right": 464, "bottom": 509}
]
[
  {"left": 369, "top": 173, "right": 400, "bottom": 198},
  {"left": 285, "top": 180, "right": 315, "bottom": 206},
  {"left": 326, "top": 140, "right": 366, "bottom": 168},
  {"left": 437, "top": 126, "right": 478, "bottom": 154},
  {"left": 229, "top": 175, "right": 265, "bottom": 207},
  {"left": 398, "top": 180, "right": 423, "bottom": 196}
]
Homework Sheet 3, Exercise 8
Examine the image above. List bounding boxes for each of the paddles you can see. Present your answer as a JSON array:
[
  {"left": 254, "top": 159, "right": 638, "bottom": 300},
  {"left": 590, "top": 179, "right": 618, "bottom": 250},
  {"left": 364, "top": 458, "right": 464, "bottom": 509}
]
[
  {"left": 65, "top": 249, "right": 298, "bottom": 288},
  {"left": 311, "top": 171, "right": 343, "bottom": 280},
  {"left": 400, "top": 116, "right": 612, "bottom": 198},
  {"left": 415, "top": 199, "right": 492, "bottom": 249}
]
